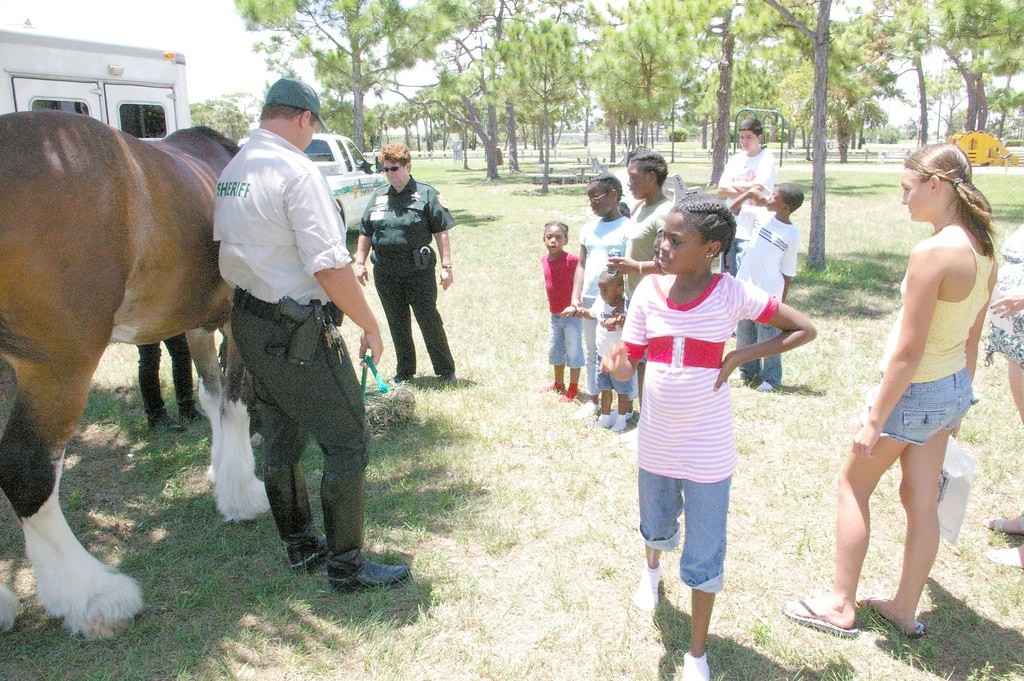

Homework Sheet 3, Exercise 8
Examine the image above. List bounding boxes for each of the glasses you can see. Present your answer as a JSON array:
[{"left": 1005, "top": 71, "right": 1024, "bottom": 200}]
[
  {"left": 588, "top": 189, "right": 612, "bottom": 203},
  {"left": 384, "top": 162, "right": 404, "bottom": 173}
]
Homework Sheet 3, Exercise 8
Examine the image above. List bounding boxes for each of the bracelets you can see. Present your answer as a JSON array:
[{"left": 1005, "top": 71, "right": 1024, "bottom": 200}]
[{"left": 639, "top": 261, "right": 643, "bottom": 275}]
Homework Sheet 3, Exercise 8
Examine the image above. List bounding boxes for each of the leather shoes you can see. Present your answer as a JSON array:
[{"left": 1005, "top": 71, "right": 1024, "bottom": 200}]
[
  {"left": 328, "top": 554, "right": 409, "bottom": 595},
  {"left": 288, "top": 537, "right": 331, "bottom": 573}
]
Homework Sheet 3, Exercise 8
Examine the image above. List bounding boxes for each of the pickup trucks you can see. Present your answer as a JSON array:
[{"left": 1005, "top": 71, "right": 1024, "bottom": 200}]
[{"left": 237, "top": 134, "right": 386, "bottom": 247}]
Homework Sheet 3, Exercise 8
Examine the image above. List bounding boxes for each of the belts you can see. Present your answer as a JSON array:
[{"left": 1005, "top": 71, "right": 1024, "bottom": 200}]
[{"left": 235, "top": 288, "right": 281, "bottom": 321}]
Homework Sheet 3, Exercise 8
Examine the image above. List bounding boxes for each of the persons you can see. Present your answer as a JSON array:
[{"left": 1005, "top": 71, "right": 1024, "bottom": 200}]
[
  {"left": 572, "top": 173, "right": 634, "bottom": 426},
  {"left": 212, "top": 80, "right": 410, "bottom": 591},
  {"left": 541, "top": 223, "right": 584, "bottom": 403},
  {"left": 982, "top": 230, "right": 1024, "bottom": 567},
  {"left": 730, "top": 184, "right": 804, "bottom": 390},
  {"left": 599, "top": 195, "right": 818, "bottom": 681},
  {"left": 355, "top": 142, "right": 457, "bottom": 386},
  {"left": 718, "top": 119, "right": 776, "bottom": 336},
  {"left": 606, "top": 147, "right": 679, "bottom": 423},
  {"left": 783, "top": 144, "right": 998, "bottom": 640},
  {"left": 137, "top": 332, "right": 208, "bottom": 433},
  {"left": 561, "top": 271, "right": 633, "bottom": 431}
]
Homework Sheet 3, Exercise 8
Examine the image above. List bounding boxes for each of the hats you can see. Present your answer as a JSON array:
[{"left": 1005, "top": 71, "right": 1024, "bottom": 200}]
[{"left": 265, "top": 78, "right": 329, "bottom": 133}]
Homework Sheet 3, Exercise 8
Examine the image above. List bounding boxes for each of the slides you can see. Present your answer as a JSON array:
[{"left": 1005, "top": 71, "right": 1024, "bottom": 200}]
[{"left": 995, "top": 140, "right": 1018, "bottom": 165}]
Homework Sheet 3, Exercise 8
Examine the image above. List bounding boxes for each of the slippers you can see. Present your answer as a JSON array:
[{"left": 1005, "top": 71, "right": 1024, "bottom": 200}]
[
  {"left": 782, "top": 601, "right": 860, "bottom": 638},
  {"left": 982, "top": 516, "right": 1024, "bottom": 534},
  {"left": 865, "top": 597, "right": 924, "bottom": 637},
  {"left": 986, "top": 546, "right": 1024, "bottom": 569}
]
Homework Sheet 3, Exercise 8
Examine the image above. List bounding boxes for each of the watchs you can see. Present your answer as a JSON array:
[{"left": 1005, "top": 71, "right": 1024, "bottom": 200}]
[{"left": 442, "top": 265, "right": 452, "bottom": 270}]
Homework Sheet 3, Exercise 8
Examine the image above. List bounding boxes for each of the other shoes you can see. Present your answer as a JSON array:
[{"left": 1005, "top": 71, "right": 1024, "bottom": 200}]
[
  {"left": 178, "top": 408, "right": 208, "bottom": 423},
  {"left": 151, "top": 414, "right": 186, "bottom": 432}
]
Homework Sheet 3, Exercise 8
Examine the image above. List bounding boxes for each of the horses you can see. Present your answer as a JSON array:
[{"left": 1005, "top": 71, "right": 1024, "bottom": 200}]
[{"left": 0, "top": 109, "right": 270, "bottom": 640}]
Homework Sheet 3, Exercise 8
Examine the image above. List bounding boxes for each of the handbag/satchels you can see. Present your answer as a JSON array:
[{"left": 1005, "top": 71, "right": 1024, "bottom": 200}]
[{"left": 938, "top": 435, "right": 975, "bottom": 545}]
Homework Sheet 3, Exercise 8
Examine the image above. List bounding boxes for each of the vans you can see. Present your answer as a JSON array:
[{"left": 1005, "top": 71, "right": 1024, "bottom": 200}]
[{"left": 0, "top": 29, "right": 189, "bottom": 142}]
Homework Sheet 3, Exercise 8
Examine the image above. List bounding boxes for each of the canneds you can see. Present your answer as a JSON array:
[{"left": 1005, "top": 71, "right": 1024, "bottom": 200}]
[{"left": 607, "top": 248, "right": 622, "bottom": 274}]
